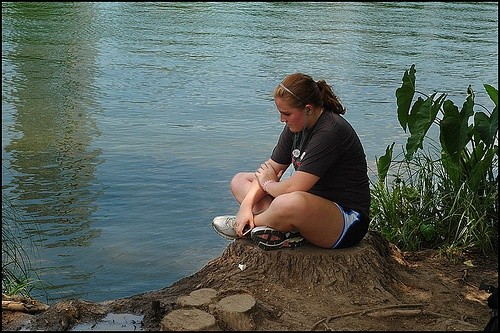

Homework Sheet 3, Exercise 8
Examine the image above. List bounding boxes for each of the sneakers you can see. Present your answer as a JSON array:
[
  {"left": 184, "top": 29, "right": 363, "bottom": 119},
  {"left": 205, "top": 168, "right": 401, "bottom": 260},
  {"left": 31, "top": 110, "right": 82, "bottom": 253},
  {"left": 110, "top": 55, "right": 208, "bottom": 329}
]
[
  {"left": 251, "top": 225, "right": 305, "bottom": 250},
  {"left": 212, "top": 215, "right": 251, "bottom": 239}
]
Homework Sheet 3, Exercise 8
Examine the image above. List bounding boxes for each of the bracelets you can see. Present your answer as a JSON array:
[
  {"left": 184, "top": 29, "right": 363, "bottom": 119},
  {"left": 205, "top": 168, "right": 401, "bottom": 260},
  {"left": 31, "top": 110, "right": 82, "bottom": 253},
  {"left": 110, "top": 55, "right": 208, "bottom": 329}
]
[{"left": 263, "top": 179, "right": 276, "bottom": 190}]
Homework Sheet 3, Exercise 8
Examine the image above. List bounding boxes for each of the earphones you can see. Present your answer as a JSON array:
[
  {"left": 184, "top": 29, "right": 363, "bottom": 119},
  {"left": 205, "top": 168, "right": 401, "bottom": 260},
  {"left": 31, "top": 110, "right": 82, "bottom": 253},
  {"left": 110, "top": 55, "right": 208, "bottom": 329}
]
[{"left": 306, "top": 108, "right": 310, "bottom": 112}]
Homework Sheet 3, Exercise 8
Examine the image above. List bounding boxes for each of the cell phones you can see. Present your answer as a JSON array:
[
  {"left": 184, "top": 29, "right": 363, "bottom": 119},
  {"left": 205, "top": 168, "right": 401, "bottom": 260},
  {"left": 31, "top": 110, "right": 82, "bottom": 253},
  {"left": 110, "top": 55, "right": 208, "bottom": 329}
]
[{"left": 243, "top": 224, "right": 251, "bottom": 236}]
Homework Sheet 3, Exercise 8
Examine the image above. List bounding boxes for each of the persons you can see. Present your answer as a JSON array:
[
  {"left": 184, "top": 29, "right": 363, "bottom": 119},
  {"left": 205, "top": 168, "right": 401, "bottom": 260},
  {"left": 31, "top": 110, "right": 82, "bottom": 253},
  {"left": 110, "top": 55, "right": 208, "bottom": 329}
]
[{"left": 212, "top": 72, "right": 371, "bottom": 251}]
